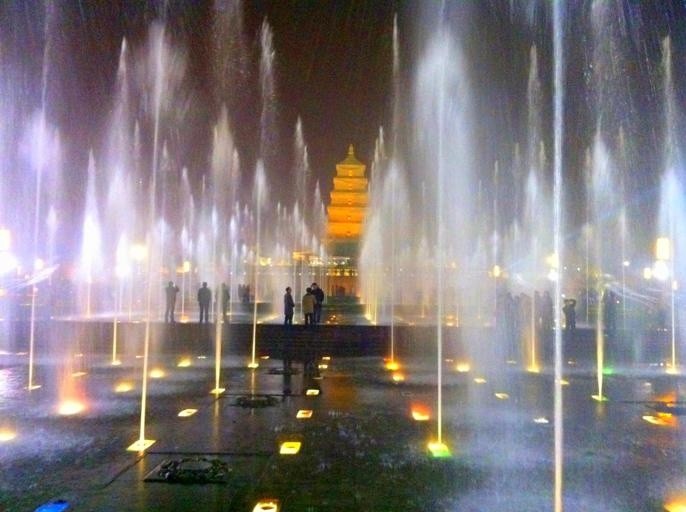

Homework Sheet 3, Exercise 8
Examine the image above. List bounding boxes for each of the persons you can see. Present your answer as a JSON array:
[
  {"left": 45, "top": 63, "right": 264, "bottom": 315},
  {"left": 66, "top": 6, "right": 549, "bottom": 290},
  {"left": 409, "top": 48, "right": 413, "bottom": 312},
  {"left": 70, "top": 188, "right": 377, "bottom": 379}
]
[
  {"left": 247, "top": 285, "right": 250, "bottom": 301},
  {"left": 238, "top": 284, "right": 242, "bottom": 302},
  {"left": 606, "top": 292, "right": 618, "bottom": 331},
  {"left": 214, "top": 282, "right": 230, "bottom": 323},
  {"left": 198, "top": 281, "right": 212, "bottom": 324},
  {"left": 331, "top": 285, "right": 345, "bottom": 296},
  {"left": 242, "top": 284, "right": 247, "bottom": 302},
  {"left": 301, "top": 287, "right": 317, "bottom": 325},
  {"left": 495, "top": 288, "right": 577, "bottom": 335},
  {"left": 164, "top": 280, "right": 180, "bottom": 324},
  {"left": 310, "top": 282, "right": 325, "bottom": 322},
  {"left": 282, "top": 286, "right": 295, "bottom": 326}
]
